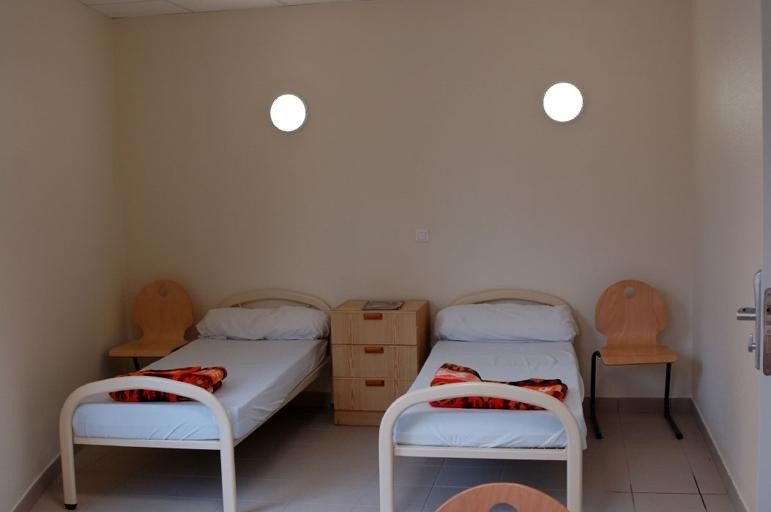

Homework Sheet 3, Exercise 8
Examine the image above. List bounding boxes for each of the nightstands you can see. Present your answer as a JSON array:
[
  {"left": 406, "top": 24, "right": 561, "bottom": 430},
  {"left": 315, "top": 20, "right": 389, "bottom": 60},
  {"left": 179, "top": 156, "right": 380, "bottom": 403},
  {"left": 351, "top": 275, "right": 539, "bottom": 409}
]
[{"left": 328, "top": 299, "right": 429, "bottom": 426}]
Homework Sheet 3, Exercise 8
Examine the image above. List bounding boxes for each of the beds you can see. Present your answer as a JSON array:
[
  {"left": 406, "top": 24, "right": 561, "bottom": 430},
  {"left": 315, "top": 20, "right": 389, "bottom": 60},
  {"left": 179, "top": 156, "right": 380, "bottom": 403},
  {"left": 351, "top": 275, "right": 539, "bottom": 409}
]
[
  {"left": 379, "top": 289, "right": 583, "bottom": 511},
  {"left": 59, "top": 288, "right": 330, "bottom": 512}
]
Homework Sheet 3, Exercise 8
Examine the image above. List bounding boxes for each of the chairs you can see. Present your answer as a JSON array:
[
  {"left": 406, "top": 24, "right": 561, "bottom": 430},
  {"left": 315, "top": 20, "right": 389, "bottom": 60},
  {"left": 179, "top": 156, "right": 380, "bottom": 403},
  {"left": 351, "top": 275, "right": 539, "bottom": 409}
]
[
  {"left": 589, "top": 279, "right": 683, "bottom": 440},
  {"left": 434, "top": 482, "right": 568, "bottom": 512},
  {"left": 109, "top": 278, "right": 194, "bottom": 372}
]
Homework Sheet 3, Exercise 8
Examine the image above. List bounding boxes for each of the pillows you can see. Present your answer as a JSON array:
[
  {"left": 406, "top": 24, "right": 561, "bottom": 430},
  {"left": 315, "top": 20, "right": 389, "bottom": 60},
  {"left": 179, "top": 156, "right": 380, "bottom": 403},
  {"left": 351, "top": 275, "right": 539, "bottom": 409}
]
[
  {"left": 194, "top": 306, "right": 328, "bottom": 339},
  {"left": 435, "top": 304, "right": 579, "bottom": 341}
]
